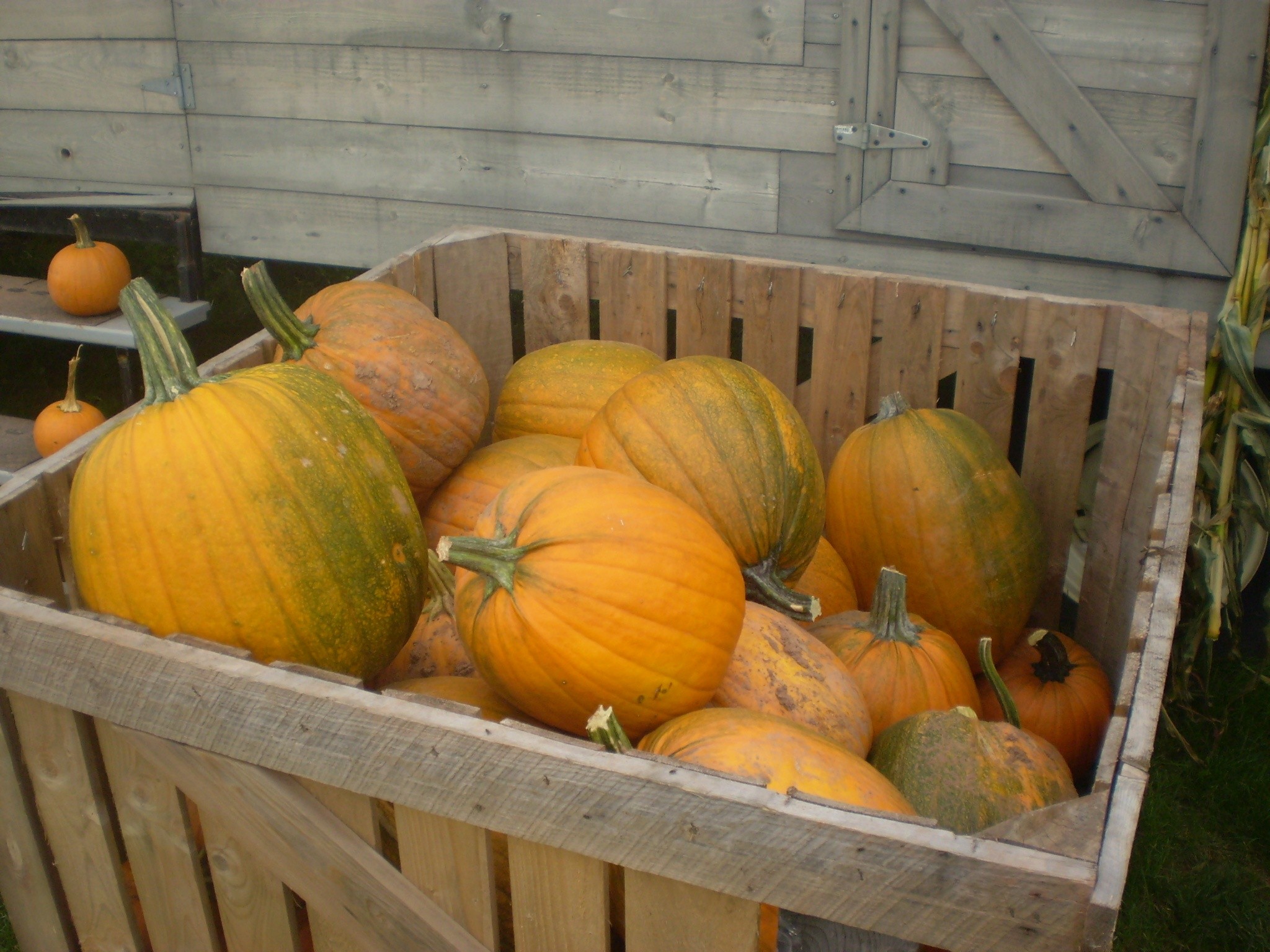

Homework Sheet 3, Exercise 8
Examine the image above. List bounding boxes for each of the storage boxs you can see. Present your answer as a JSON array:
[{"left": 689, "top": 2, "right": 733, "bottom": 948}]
[{"left": 0, "top": 228, "right": 1209, "bottom": 951}]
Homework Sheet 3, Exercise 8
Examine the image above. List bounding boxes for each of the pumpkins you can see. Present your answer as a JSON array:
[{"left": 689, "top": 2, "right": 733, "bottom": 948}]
[
  {"left": 47, "top": 216, "right": 130, "bottom": 316},
  {"left": 34, "top": 359, "right": 105, "bottom": 456},
  {"left": 71, "top": 263, "right": 1116, "bottom": 952}
]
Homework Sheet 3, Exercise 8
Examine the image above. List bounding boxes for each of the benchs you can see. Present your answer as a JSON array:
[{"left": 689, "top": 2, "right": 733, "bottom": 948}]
[{"left": 0, "top": 272, "right": 211, "bottom": 408}]
